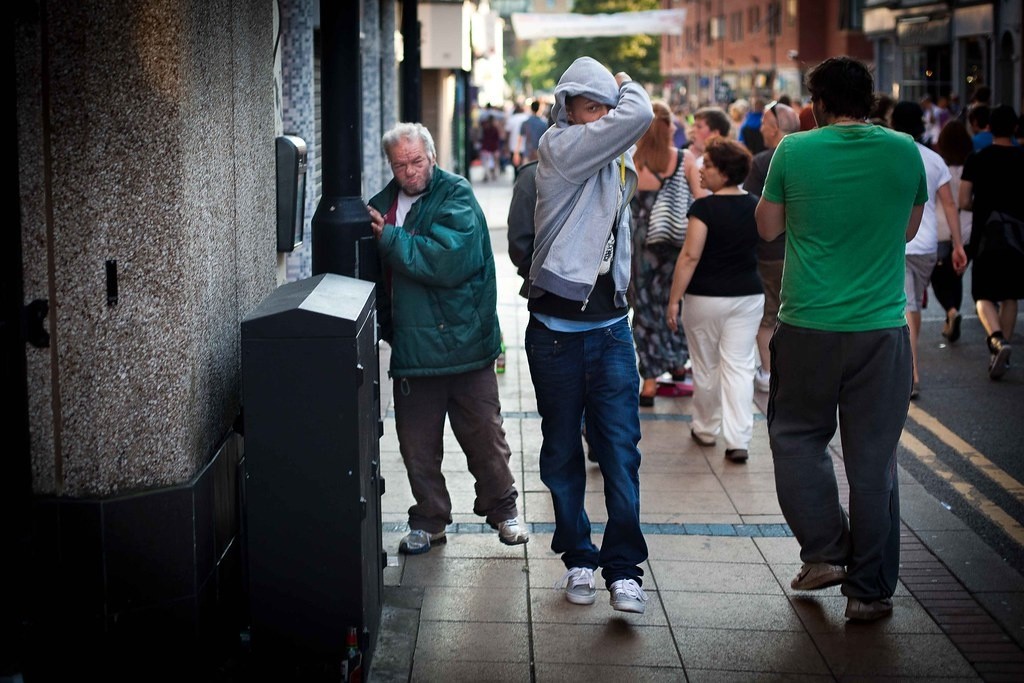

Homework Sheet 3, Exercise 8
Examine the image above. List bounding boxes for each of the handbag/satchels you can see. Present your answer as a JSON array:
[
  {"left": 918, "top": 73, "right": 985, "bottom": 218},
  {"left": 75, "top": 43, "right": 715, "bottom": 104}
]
[{"left": 645, "top": 155, "right": 694, "bottom": 253}]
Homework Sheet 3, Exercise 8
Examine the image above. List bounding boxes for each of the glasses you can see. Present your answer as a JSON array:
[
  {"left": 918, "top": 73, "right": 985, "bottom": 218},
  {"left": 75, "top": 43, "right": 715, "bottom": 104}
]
[{"left": 765, "top": 101, "right": 778, "bottom": 117}]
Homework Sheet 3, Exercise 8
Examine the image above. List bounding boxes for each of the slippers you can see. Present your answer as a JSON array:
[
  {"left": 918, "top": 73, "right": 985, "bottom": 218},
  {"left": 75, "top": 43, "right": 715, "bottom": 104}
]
[
  {"left": 671, "top": 368, "right": 686, "bottom": 381},
  {"left": 639, "top": 393, "right": 654, "bottom": 406}
]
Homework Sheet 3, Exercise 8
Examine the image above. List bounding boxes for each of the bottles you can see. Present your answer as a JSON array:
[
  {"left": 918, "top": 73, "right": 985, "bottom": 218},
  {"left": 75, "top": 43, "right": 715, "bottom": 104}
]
[{"left": 496, "top": 336, "right": 505, "bottom": 373}]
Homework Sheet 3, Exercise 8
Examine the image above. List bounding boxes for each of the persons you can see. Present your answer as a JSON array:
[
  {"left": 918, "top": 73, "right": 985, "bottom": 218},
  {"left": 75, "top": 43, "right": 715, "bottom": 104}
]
[
  {"left": 471, "top": 56, "right": 1024, "bottom": 620},
  {"left": 366, "top": 121, "right": 529, "bottom": 554}
]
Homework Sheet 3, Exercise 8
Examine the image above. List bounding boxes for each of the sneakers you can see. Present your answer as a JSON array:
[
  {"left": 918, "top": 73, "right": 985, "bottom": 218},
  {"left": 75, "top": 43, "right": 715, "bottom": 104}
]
[
  {"left": 398, "top": 529, "right": 447, "bottom": 554},
  {"left": 609, "top": 578, "right": 649, "bottom": 613},
  {"left": 845, "top": 598, "right": 894, "bottom": 621},
  {"left": 491, "top": 516, "right": 529, "bottom": 545},
  {"left": 791, "top": 562, "right": 846, "bottom": 591},
  {"left": 553, "top": 567, "right": 598, "bottom": 604},
  {"left": 987, "top": 331, "right": 1013, "bottom": 381}
]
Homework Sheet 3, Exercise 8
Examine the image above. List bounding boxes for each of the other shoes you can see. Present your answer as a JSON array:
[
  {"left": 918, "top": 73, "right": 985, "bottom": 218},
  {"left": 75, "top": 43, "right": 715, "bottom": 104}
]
[
  {"left": 910, "top": 384, "right": 920, "bottom": 399},
  {"left": 754, "top": 365, "right": 771, "bottom": 392},
  {"left": 724, "top": 449, "right": 748, "bottom": 461},
  {"left": 942, "top": 313, "right": 962, "bottom": 343},
  {"left": 690, "top": 428, "right": 716, "bottom": 447}
]
